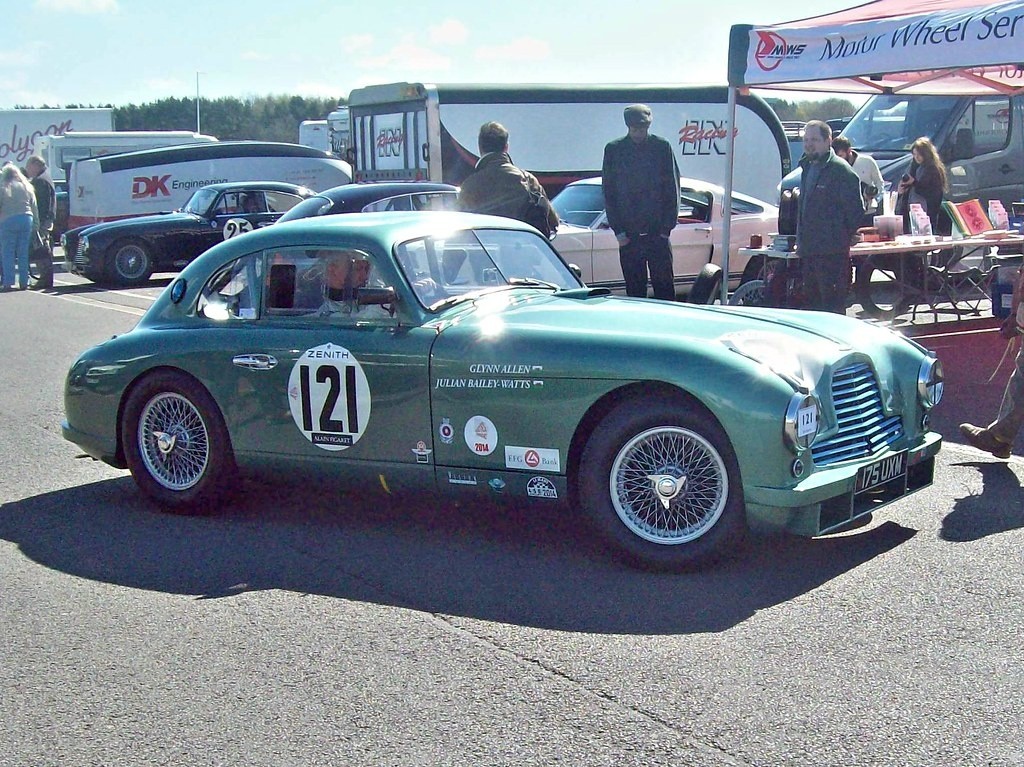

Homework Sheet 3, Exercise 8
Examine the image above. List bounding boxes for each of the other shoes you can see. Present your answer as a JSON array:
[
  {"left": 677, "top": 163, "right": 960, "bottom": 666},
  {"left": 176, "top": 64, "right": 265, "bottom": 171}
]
[
  {"left": 41, "top": 286, "right": 53, "bottom": 293},
  {"left": 30, "top": 282, "right": 46, "bottom": 289},
  {"left": 0, "top": 284, "right": 11, "bottom": 292},
  {"left": 961, "top": 423, "right": 1010, "bottom": 459},
  {"left": 20, "top": 285, "right": 28, "bottom": 291}
]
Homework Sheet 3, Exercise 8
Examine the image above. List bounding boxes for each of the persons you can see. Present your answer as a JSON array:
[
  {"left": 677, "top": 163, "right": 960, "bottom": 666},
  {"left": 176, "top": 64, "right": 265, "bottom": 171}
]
[
  {"left": 441, "top": 121, "right": 559, "bottom": 286},
  {"left": 602, "top": 104, "right": 681, "bottom": 301},
  {"left": 796, "top": 120, "right": 866, "bottom": 316},
  {"left": 304, "top": 248, "right": 440, "bottom": 318},
  {"left": 832, "top": 137, "right": 884, "bottom": 228},
  {"left": 0, "top": 156, "right": 56, "bottom": 293},
  {"left": 958, "top": 263, "right": 1024, "bottom": 459},
  {"left": 898, "top": 136, "right": 948, "bottom": 235}
]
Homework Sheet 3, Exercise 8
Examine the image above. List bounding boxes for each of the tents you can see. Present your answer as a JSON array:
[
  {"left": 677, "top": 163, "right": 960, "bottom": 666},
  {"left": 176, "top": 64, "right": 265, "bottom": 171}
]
[{"left": 721, "top": 0, "right": 1024, "bottom": 305}]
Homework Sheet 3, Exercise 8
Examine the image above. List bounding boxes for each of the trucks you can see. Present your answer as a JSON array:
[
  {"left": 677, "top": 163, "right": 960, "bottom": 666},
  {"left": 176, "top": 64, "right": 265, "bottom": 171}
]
[
  {"left": 0, "top": 106, "right": 353, "bottom": 247},
  {"left": 344, "top": 79, "right": 792, "bottom": 206}
]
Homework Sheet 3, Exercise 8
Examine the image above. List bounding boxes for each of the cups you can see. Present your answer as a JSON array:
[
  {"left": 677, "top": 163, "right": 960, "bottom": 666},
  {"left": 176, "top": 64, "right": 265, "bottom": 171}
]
[{"left": 751, "top": 234, "right": 762, "bottom": 247}]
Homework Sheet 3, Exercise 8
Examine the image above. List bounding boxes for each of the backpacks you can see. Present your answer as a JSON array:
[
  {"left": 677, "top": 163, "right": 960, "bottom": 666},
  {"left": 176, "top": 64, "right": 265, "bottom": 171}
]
[{"left": 518, "top": 172, "right": 550, "bottom": 241}]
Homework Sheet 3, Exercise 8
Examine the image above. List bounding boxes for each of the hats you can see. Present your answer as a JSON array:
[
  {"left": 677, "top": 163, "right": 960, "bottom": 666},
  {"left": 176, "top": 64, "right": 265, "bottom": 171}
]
[{"left": 624, "top": 104, "right": 652, "bottom": 128}]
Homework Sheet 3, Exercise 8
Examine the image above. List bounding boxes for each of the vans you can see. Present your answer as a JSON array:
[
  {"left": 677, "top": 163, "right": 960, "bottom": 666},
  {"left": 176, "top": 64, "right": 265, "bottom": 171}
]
[{"left": 778, "top": 91, "right": 1024, "bottom": 235}]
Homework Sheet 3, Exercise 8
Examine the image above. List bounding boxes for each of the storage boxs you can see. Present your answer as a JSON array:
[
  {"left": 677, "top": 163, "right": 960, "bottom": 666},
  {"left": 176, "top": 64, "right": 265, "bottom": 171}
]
[{"left": 857, "top": 226, "right": 880, "bottom": 242}]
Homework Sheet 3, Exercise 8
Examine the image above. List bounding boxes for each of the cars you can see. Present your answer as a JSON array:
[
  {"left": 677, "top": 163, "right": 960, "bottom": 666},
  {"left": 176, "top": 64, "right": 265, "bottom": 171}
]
[
  {"left": 778, "top": 97, "right": 1010, "bottom": 171},
  {"left": 542, "top": 176, "right": 780, "bottom": 288},
  {"left": 56, "top": 209, "right": 946, "bottom": 572},
  {"left": 266, "top": 182, "right": 460, "bottom": 289},
  {"left": 60, "top": 180, "right": 319, "bottom": 287}
]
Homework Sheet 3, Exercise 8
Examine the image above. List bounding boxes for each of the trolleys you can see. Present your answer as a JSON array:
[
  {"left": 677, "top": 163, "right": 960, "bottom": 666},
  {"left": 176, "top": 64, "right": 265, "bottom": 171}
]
[{"left": 727, "top": 225, "right": 1024, "bottom": 321}]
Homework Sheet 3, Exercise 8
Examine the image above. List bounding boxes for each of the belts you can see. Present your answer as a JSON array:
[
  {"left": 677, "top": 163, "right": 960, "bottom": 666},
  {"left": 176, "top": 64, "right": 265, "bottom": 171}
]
[{"left": 628, "top": 233, "right": 656, "bottom": 236}]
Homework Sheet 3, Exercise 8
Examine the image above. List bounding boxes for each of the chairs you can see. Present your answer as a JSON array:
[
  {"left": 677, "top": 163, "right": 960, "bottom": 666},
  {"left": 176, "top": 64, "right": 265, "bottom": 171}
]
[
  {"left": 235, "top": 196, "right": 255, "bottom": 213},
  {"left": 947, "top": 127, "right": 975, "bottom": 161},
  {"left": 268, "top": 264, "right": 297, "bottom": 308},
  {"left": 923, "top": 247, "right": 992, "bottom": 311}
]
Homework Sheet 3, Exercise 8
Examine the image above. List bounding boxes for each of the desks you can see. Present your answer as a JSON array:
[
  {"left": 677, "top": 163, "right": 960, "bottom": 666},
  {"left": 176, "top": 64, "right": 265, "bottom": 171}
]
[{"left": 737, "top": 234, "right": 1024, "bottom": 329}]
